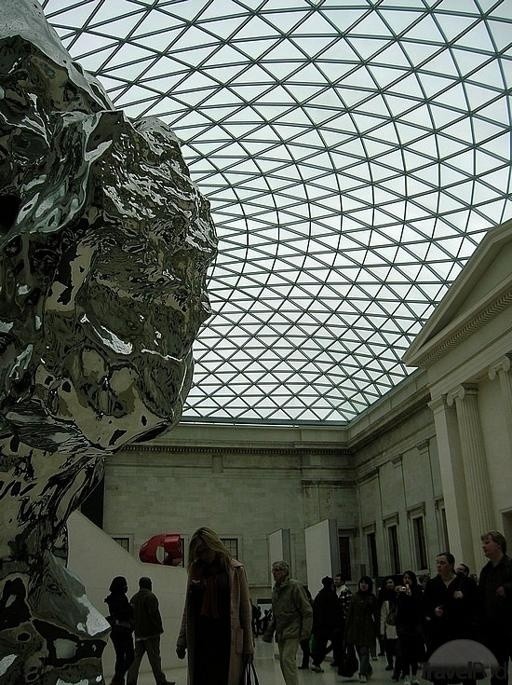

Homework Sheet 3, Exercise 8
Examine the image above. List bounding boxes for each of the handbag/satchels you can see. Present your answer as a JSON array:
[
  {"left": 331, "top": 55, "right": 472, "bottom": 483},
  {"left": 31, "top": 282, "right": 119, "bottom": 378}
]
[{"left": 338, "top": 653, "right": 359, "bottom": 677}]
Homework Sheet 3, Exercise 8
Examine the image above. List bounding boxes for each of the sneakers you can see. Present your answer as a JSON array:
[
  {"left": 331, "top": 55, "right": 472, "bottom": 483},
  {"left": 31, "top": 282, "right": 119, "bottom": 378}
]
[
  {"left": 385, "top": 664, "right": 422, "bottom": 685},
  {"left": 298, "top": 661, "right": 325, "bottom": 673},
  {"left": 360, "top": 674, "right": 366, "bottom": 682}
]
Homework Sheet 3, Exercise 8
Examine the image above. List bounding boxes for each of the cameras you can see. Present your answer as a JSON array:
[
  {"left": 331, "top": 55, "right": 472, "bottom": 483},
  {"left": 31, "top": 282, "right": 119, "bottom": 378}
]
[{"left": 398, "top": 587, "right": 407, "bottom": 592}]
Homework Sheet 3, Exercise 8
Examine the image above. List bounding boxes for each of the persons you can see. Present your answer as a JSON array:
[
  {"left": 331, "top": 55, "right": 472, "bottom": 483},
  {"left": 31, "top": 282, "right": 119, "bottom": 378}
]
[
  {"left": 104, "top": 577, "right": 133, "bottom": 685},
  {"left": 300, "top": 530, "right": 512, "bottom": 684},
  {"left": 250, "top": 598, "right": 272, "bottom": 637},
  {"left": 127, "top": 577, "right": 176, "bottom": 685},
  {"left": 263, "top": 560, "right": 314, "bottom": 685},
  {"left": 176, "top": 527, "right": 254, "bottom": 685}
]
